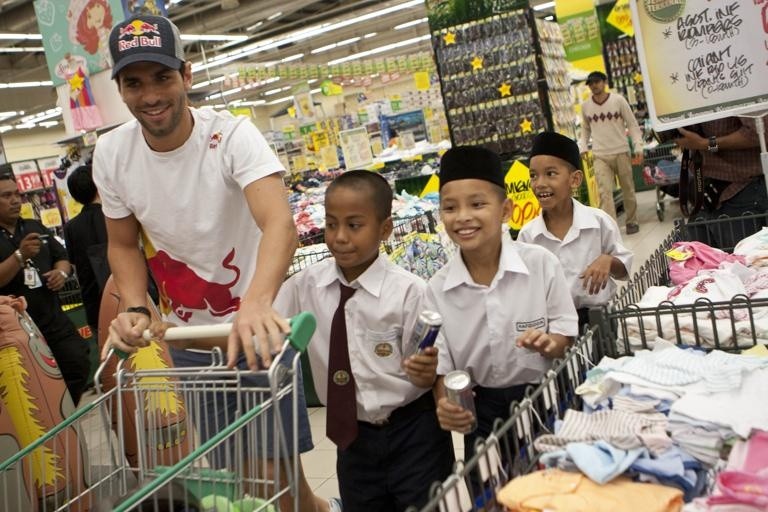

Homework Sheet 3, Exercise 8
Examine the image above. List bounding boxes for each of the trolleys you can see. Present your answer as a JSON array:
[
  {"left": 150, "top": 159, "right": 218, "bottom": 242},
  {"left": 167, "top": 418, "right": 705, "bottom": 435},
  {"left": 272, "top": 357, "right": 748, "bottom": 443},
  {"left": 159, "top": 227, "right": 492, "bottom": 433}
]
[
  {"left": 1, "top": 312, "right": 315, "bottom": 512},
  {"left": 640, "top": 143, "right": 682, "bottom": 220}
]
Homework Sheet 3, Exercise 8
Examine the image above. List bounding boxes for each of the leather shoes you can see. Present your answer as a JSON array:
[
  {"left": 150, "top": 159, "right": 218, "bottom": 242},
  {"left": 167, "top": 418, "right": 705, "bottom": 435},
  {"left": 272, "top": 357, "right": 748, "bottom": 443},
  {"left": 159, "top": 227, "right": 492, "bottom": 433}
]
[{"left": 626, "top": 222, "right": 640, "bottom": 235}]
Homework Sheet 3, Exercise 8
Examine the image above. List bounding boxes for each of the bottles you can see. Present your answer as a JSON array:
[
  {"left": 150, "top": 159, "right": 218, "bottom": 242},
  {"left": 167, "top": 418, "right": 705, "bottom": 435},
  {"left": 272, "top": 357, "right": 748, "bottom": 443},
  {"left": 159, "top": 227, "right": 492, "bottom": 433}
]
[
  {"left": 444, "top": 370, "right": 479, "bottom": 437},
  {"left": 400, "top": 311, "right": 443, "bottom": 370}
]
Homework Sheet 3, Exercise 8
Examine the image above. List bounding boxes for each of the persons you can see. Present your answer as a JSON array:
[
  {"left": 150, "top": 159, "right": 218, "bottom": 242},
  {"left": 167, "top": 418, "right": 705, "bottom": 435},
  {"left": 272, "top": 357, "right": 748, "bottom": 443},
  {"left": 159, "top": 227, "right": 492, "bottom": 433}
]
[
  {"left": 679, "top": 112, "right": 767, "bottom": 255},
  {"left": 0, "top": 168, "right": 91, "bottom": 395},
  {"left": 63, "top": 166, "right": 106, "bottom": 332},
  {"left": 579, "top": 71, "right": 644, "bottom": 235},
  {"left": 387, "top": 128, "right": 400, "bottom": 148},
  {"left": 426, "top": 148, "right": 578, "bottom": 499},
  {"left": 272, "top": 170, "right": 454, "bottom": 512},
  {"left": 520, "top": 133, "right": 632, "bottom": 341},
  {"left": 93, "top": 15, "right": 338, "bottom": 512}
]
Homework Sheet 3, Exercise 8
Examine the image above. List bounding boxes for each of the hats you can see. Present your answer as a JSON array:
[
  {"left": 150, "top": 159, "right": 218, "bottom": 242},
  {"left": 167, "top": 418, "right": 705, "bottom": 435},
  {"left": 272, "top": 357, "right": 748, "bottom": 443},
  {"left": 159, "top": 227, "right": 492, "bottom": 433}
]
[
  {"left": 585, "top": 71, "right": 607, "bottom": 85},
  {"left": 106, "top": 13, "right": 186, "bottom": 80},
  {"left": 529, "top": 131, "right": 580, "bottom": 169},
  {"left": 439, "top": 145, "right": 505, "bottom": 191}
]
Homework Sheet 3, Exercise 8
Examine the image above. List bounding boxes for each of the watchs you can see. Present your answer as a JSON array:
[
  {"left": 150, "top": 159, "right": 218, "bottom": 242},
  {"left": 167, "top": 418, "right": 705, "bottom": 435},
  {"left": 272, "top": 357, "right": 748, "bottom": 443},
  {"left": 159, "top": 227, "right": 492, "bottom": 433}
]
[
  {"left": 127, "top": 305, "right": 151, "bottom": 316},
  {"left": 56, "top": 269, "right": 68, "bottom": 278},
  {"left": 707, "top": 135, "right": 719, "bottom": 152}
]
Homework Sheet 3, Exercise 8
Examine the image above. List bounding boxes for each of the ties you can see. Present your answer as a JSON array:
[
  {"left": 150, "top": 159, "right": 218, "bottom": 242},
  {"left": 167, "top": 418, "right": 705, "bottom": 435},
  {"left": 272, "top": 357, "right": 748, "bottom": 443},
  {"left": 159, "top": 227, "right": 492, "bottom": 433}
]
[{"left": 325, "top": 284, "right": 358, "bottom": 452}]
